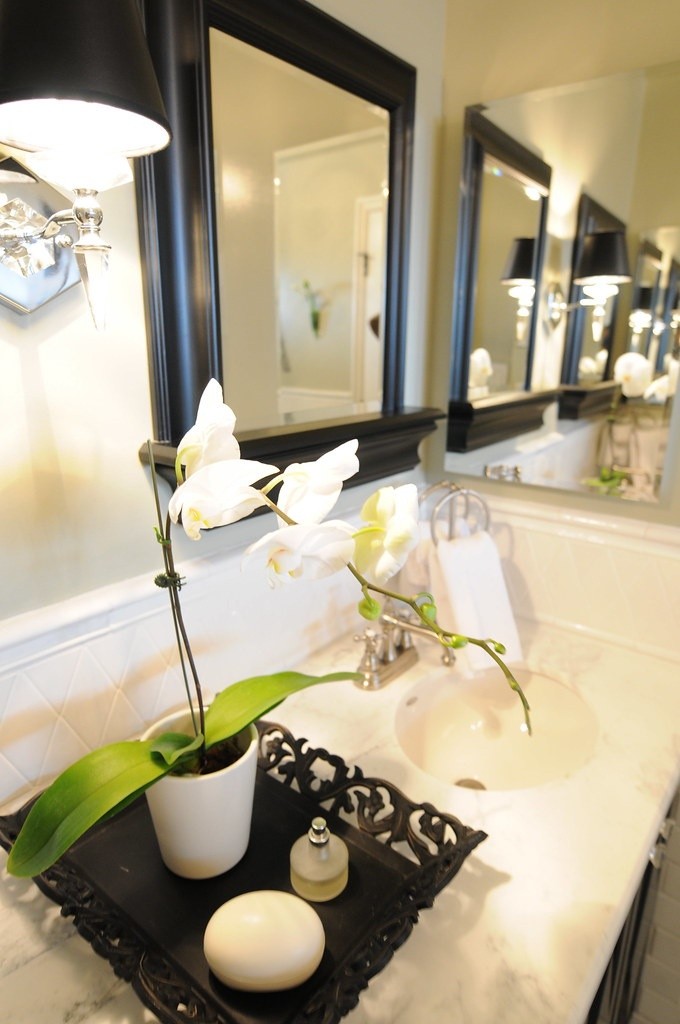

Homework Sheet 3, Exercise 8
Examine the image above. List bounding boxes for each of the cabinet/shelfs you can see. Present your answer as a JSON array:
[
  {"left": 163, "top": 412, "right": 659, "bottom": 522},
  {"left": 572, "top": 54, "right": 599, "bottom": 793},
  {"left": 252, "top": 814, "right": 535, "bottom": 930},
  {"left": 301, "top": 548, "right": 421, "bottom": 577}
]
[{"left": 583, "top": 792, "right": 678, "bottom": 1024}]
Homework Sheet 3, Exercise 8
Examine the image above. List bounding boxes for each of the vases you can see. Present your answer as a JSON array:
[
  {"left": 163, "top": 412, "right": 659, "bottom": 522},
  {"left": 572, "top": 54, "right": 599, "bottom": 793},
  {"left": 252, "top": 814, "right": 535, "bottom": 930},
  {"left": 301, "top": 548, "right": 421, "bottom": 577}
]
[{"left": 139, "top": 705, "right": 260, "bottom": 881}]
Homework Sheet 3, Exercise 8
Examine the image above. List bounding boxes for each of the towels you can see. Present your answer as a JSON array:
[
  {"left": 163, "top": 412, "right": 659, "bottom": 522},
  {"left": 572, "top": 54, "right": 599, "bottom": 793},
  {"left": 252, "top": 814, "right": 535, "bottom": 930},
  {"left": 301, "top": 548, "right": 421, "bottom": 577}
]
[{"left": 428, "top": 527, "right": 521, "bottom": 669}]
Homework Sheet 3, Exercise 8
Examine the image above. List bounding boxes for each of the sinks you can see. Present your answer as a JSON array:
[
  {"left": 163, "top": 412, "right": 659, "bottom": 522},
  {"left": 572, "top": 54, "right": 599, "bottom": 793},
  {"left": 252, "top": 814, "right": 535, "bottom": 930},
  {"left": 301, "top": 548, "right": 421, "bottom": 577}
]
[{"left": 392, "top": 663, "right": 603, "bottom": 791}]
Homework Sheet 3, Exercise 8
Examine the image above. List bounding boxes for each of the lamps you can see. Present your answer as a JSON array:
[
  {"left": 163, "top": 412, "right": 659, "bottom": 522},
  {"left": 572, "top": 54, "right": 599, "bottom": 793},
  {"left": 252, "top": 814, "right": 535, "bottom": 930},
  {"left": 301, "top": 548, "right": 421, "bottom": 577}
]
[
  {"left": 541, "top": 229, "right": 633, "bottom": 342},
  {"left": 627, "top": 284, "right": 680, "bottom": 338},
  {"left": 498, "top": 236, "right": 537, "bottom": 344},
  {"left": 0, "top": 0, "right": 174, "bottom": 317}
]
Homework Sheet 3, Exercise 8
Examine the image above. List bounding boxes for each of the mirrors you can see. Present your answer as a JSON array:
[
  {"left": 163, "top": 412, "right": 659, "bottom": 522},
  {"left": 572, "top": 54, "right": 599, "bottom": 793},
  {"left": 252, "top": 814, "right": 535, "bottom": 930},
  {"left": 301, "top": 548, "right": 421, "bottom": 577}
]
[
  {"left": 654, "top": 257, "right": 680, "bottom": 380},
  {"left": 445, "top": 57, "right": 680, "bottom": 511},
  {"left": 132, "top": 1, "right": 448, "bottom": 531},
  {"left": 619, "top": 235, "right": 671, "bottom": 406},
  {"left": 444, "top": 107, "right": 564, "bottom": 455},
  {"left": 554, "top": 192, "right": 629, "bottom": 421}
]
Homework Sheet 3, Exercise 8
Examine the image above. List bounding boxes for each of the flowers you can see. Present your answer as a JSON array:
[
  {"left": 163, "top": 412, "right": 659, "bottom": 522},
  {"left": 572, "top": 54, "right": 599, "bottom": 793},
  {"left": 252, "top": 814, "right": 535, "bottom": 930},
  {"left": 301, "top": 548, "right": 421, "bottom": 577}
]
[
  {"left": 466, "top": 344, "right": 679, "bottom": 504},
  {"left": 4, "top": 377, "right": 535, "bottom": 881}
]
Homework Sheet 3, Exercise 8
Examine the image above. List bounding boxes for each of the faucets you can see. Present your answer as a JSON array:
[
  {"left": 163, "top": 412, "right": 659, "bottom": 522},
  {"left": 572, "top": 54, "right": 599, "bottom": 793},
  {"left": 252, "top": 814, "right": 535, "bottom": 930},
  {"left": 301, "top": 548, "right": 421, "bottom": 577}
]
[{"left": 383, "top": 613, "right": 456, "bottom": 670}]
